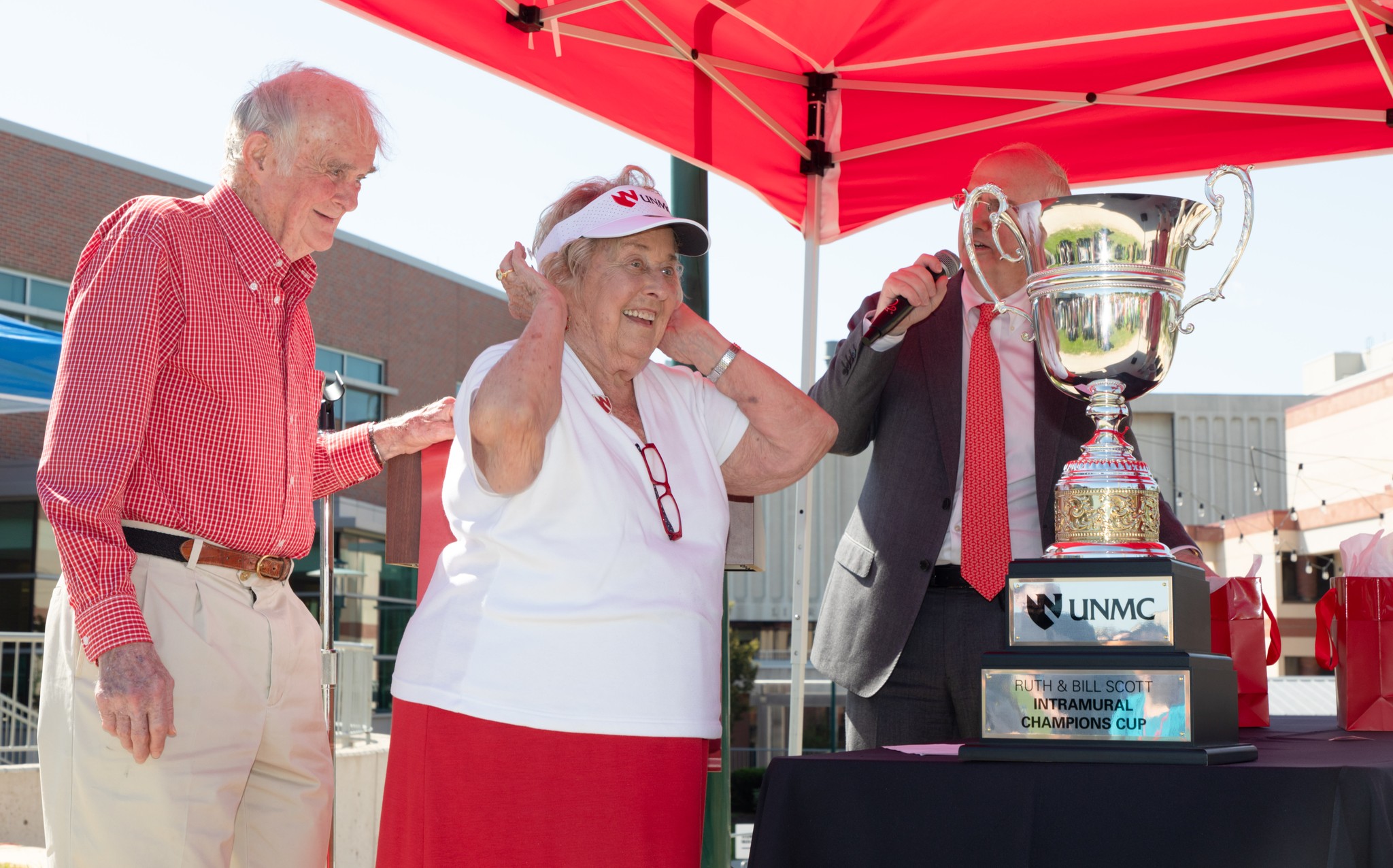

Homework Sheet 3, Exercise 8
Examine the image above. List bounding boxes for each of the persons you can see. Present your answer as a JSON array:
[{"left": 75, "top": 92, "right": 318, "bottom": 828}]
[
  {"left": 806, "top": 141, "right": 1224, "bottom": 750},
  {"left": 379, "top": 161, "right": 839, "bottom": 867},
  {"left": 35, "top": 63, "right": 455, "bottom": 868}
]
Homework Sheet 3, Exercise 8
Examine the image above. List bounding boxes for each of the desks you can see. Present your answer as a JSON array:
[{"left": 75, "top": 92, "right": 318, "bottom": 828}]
[{"left": 748, "top": 715, "right": 1393, "bottom": 868}]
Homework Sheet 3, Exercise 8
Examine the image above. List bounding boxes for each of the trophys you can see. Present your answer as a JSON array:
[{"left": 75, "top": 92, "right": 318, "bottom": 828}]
[{"left": 957, "top": 163, "right": 1260, "bottom": 767}]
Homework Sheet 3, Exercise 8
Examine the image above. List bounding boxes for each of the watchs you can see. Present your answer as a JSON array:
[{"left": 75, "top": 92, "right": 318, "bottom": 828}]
[
  {"left": 368, "top": 420, "right": 387, "bottom": 466},
  {"left": 1189, "top": 548, "right": 1200, "bottom": 556}
]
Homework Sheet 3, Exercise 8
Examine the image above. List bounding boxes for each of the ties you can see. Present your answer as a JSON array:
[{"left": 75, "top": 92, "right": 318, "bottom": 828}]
[{"left": 960, "top": 301, "right": 1011, "bottom": 603}]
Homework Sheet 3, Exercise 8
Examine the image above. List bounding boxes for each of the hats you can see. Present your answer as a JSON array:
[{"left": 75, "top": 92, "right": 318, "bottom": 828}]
[{"left": 534, "top": 185, "right": 712, "bottom": 266}]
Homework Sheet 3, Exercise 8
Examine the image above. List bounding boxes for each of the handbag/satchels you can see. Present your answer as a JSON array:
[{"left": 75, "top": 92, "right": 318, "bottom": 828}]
[
  {"left": 1315, "top": 576, "right": 1393, "bottom": 731},
  {"left": 1205, "top": 576, "right": 1281, "bottom": 728}
]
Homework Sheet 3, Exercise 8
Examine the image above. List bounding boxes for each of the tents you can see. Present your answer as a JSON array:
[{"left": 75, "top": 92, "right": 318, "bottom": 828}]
[{"left": 320, "top": 0, "right": 1393, "bottom": 755}]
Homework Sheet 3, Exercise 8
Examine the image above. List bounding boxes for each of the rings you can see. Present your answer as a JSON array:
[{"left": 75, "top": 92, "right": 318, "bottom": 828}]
[{"left": 496, "top": 267, "right": 514, "bottom": 281}]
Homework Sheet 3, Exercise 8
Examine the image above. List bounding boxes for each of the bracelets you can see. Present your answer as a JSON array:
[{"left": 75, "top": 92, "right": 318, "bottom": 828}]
[{"left": 706, "top": 342, "right": 741, "bottom": 384}]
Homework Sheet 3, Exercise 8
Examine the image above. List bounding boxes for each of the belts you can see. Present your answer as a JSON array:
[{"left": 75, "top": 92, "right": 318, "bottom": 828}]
[
  {"left": 928, "top": 565, "right": 977, "bottom": 591},
  {"left": 122, "top": 526, "right": 292, "bottom": 581}
]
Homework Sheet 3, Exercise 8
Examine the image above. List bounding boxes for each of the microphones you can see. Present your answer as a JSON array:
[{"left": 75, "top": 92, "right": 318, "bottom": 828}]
[{"left": 861, "top": 249, "right": 960, "bottom": 348}]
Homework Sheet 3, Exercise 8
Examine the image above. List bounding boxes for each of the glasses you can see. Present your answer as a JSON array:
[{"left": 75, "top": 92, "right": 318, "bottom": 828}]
[
  {"left": 635, "top": 443, "right": 683, "bottom": 542},
  {"left": 948, "top": 193, "right": 1012, "bottom": 217}
]
